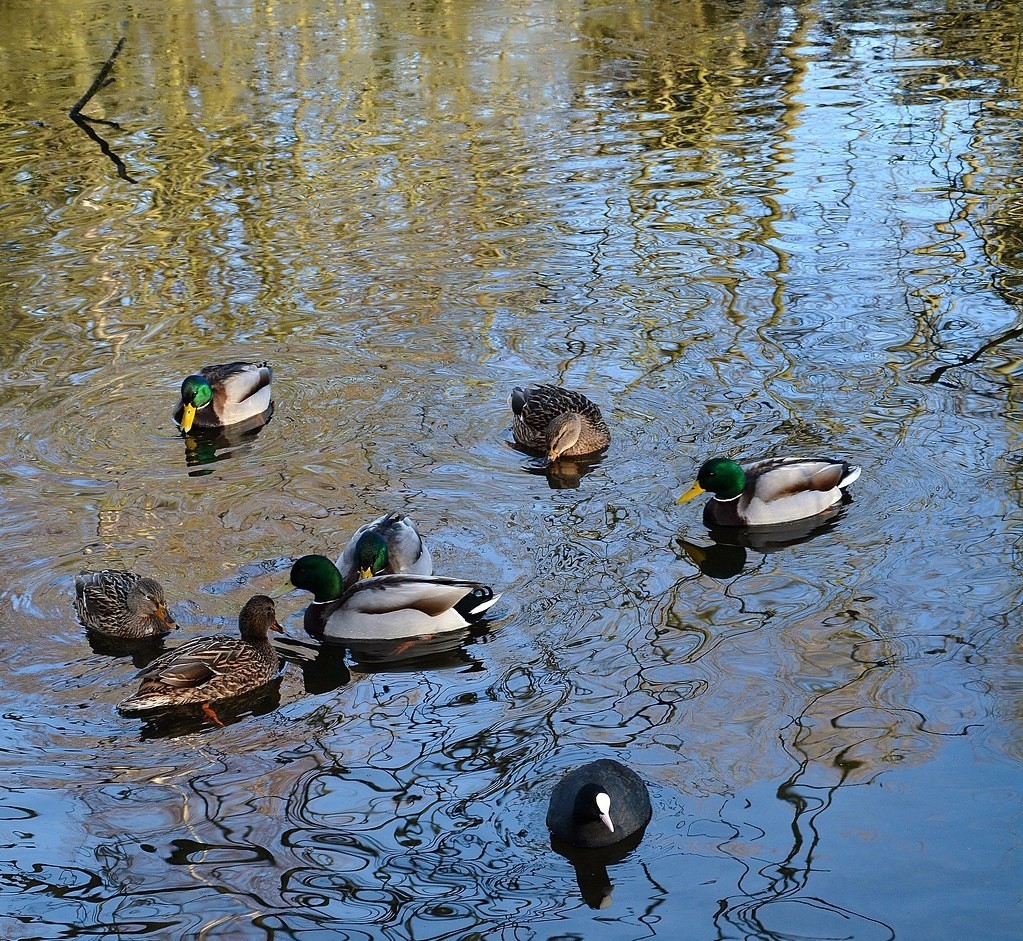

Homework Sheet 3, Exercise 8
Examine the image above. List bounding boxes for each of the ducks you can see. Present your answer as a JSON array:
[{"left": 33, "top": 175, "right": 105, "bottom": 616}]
[
  {"left": 335, "top": 511, "right": 435, "bottom": 599},
  {"left": 116, "top": 594, "right": 287, "bottom": 727},
  {"left": 544, "top": 759, "right": 651, "bottom": 850},
  {"left": 178, "top": 361, "right": 274, "bottom": 434},
  {"left": 73, "top": 567, "right": 180, "bottom": 638},
  {"left": 674, "top": 455, "right": 862, "bottom": 526},
  {"left": 567, "top": 830, "right": 648, "bottom": 911},
  {"left": 674, "top": 506, "right": 852, "bottom": 579},
  {"left": 506, "top": 382, "right": 611, "bottom": 466},
  {"left": 267, "top": 554, "right": 504, "bottom": 654}
]
